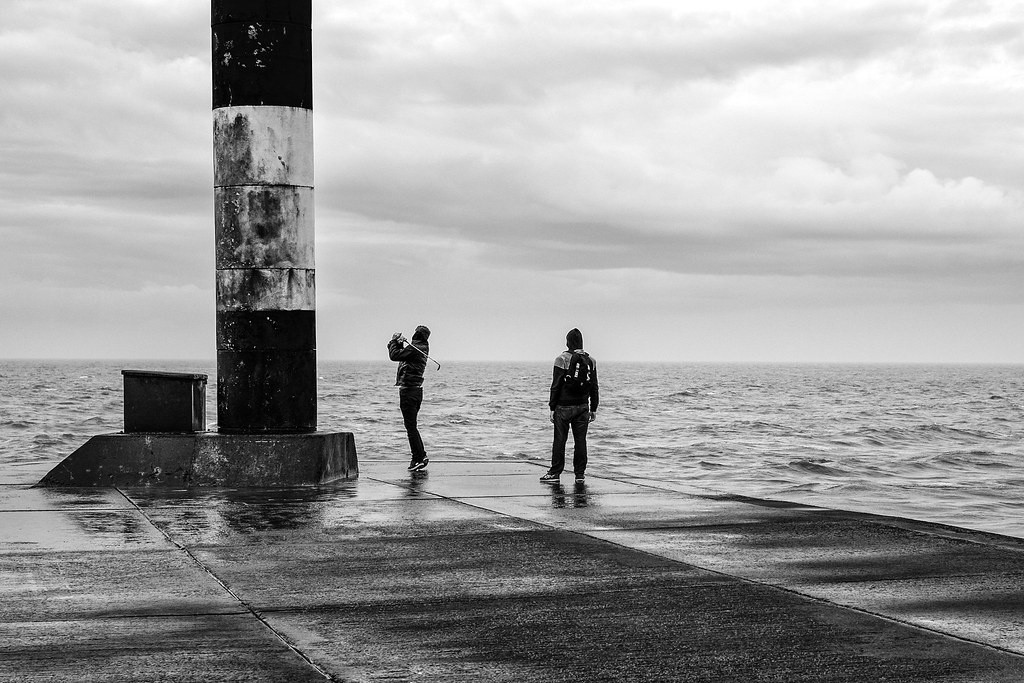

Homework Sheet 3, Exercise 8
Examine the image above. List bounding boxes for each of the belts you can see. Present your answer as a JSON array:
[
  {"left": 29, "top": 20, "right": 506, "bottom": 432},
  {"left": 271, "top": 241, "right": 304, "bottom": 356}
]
[{"left": 399, "top": 385, "right": 420, "bottom": 391}]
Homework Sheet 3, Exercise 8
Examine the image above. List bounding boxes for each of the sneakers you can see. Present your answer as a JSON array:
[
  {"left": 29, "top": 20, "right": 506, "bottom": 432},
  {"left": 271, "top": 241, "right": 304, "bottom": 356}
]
[
  {"left": 540, "top": 471, "right": 560, "bottom": 482},
  {"left": 422, "top": 455, "right": 430, "bottom": 467},
  {"left": 407, "top": 458, "right": 424, "bottom": 471},
  {"left": 575, "top": 475, "right": 585, "bottom": 484}
]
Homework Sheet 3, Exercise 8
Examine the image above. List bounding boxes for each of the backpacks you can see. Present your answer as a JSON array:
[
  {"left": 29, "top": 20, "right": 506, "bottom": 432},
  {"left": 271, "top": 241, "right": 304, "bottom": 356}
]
[{"left": 559, "top": 351, "right": 594, "bottom": 401}]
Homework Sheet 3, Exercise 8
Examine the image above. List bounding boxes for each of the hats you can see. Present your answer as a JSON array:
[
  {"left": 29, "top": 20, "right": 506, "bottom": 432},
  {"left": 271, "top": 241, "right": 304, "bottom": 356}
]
[
  {"left": 415, "top": 326, "right": 430, "bottom": 340},
  {"left": 566, "top": 328, "right": 583, "bottom": 349}
]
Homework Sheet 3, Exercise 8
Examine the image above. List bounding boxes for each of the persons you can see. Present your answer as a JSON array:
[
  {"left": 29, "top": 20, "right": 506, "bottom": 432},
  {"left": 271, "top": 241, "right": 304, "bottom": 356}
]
[
  {"left": 388, "top": 326, "right": 431, "bottom": 471},
  {"left": 540, "top": 329, "right": 600, "bottom": 482}
]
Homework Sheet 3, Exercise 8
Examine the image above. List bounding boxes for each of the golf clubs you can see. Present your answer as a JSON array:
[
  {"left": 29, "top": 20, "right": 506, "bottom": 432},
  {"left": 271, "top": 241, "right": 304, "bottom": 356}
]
[{"left": 404, "top": 340, "right": 441, "bottom": 371}]
